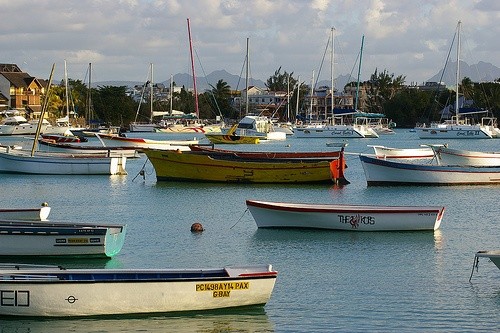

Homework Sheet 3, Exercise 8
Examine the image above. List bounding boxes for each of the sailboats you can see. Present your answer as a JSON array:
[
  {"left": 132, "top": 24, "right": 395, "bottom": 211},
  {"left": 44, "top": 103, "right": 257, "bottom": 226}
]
[
  {"left": 0, "top": 19, "right": 396, "bottom": 153},
  {"left": 413, "top": 20, "right": 500, "bottom": 140}
]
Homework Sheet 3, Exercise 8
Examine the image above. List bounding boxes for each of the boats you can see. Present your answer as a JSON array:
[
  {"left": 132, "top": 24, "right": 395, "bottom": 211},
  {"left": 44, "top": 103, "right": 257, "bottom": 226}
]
[
  {"left": 357, "top": 153, "right": 500, "bottom": 187},
  {"left": 422, "top": 143, "right": 500, "bottom": 168},
  {"left": 0, "top": 215, "right": 128, "bottom": 260},
  {"left": 0, "top": 262, "right": 279, "bottom": 319},
  {"left": 245, "top": 198, "right": 446, "bottom": 232},
  {"left": 0, "top": 143, "right": 129, "bottom": 176},
  {"left": 474, "top": 249, "right": 500, "bottom": 270},
  {"left": 134, "top": 143, "right": 353, "bottom": 187},
  {"left": 0, "top": 200, "right": 52, "bottom": 221},
  {"left": 366, "top": 144, "right": 435, "bottom": 159}
]
